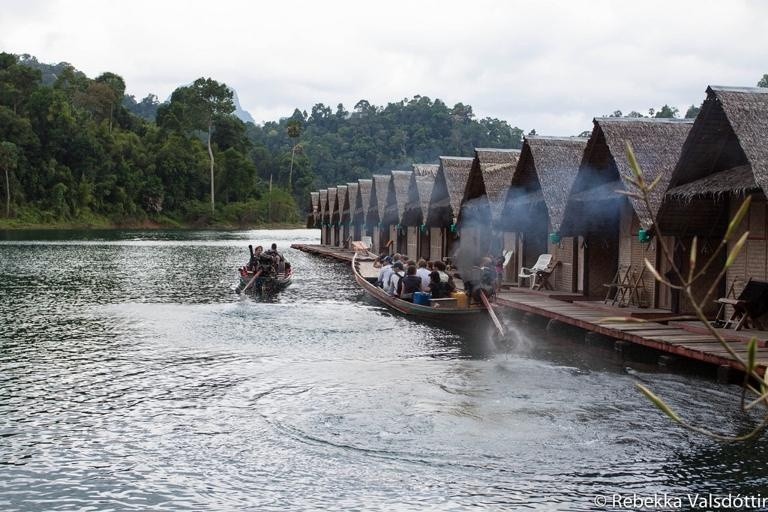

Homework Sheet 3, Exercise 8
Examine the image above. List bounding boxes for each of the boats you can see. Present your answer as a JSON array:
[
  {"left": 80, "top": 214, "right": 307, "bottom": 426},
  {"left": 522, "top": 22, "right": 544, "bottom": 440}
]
[
  {"left": 239, "top": 248, "right": 295, "bottom": 291},
  {"left": 352, "top": 240, "right": 500, "bottom": 322}
]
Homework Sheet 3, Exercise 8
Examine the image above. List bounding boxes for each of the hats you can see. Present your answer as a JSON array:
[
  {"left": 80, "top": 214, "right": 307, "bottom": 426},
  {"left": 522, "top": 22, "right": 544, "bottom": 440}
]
[
  {"left": 428, "top": 272, "right": 439, "bottom": 277},
  {"left": 391, "top": 262, "right": 403, "bottom": 268},
  {"left": 380, "top": 256, "right": 392, "bottom": 262}
]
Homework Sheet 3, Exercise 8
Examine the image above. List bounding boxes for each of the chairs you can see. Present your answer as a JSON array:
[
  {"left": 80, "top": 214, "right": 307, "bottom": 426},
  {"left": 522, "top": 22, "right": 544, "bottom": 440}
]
[
  {"left": 712, "top": 275, "right": 768, "bottom": 331},
  {"left": 602, "top": 263, "right": 646, "bottom": 308},
  {"left": 518, "top": 253, "right": 560, "bottom": 291}
]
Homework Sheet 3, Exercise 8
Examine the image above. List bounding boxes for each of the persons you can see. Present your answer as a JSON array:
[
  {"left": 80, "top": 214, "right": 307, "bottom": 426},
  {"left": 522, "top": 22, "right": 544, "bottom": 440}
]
[
  {"left": 246, "top": 242, "right": 290, "bottom": 277},
  {"left": 376, "top": 252, "right": 506, "bottom": 306}
]
